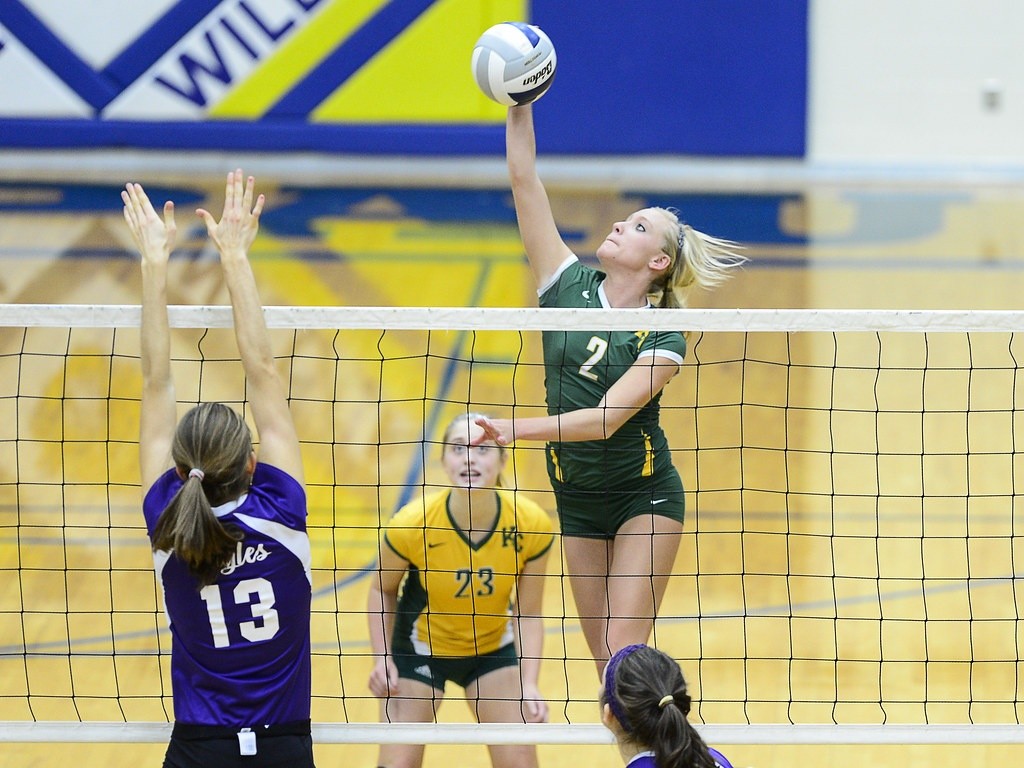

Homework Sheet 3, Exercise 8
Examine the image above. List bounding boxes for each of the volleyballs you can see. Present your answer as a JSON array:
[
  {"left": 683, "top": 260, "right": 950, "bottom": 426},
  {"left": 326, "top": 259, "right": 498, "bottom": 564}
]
[{"left": 469, "top": 19, "right": 560, "bottom": 109}]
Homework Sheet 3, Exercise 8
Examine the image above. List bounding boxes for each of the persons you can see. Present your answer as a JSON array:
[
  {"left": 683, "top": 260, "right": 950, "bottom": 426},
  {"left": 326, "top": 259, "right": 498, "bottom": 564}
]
[
  {"left": 117, "top": 163, "right": 321, "bottom": 768},
  {"left": 597, "top": 642, "right": 734, "bottom": 768},
  {"left": 467, "top": 106, "right": 751, "bottom": 707},
  {"left": 365, "top": 408, "right": 555, "bottom": 768}
]
